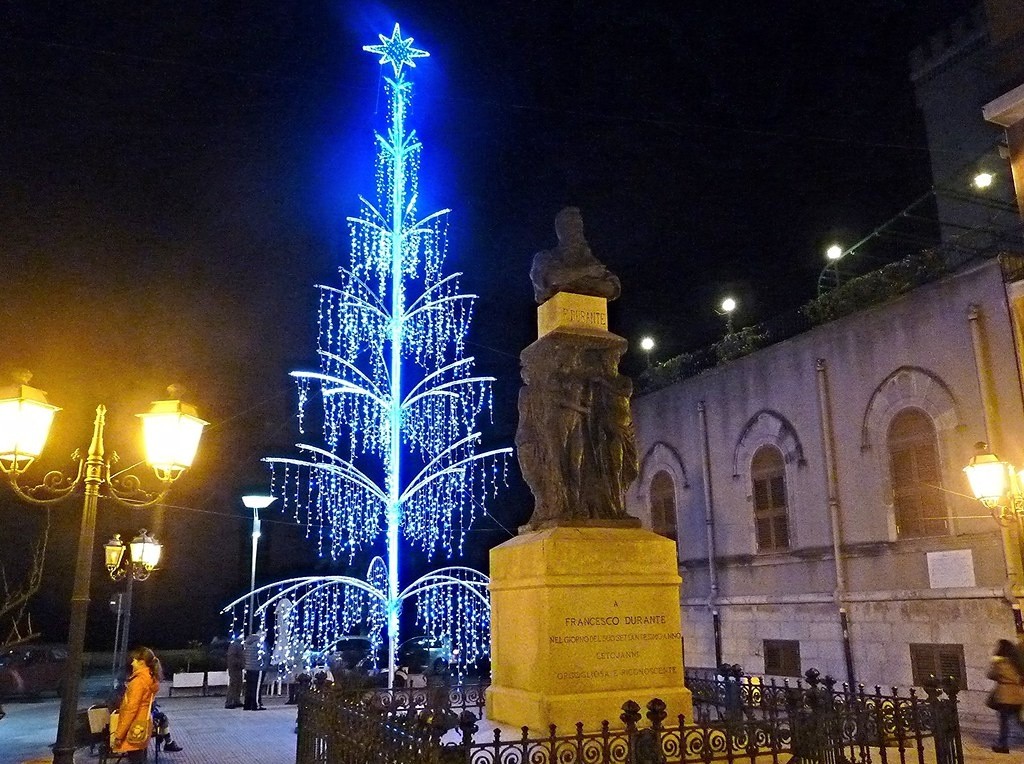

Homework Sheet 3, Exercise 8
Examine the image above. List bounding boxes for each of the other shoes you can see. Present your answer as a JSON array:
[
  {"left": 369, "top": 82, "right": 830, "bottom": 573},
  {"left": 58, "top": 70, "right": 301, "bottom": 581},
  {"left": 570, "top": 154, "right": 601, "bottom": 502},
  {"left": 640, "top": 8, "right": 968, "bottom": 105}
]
[
  {"left": 252, "top": 706, "right": 267, "bottom": 710},
  {"left": 285, "top": 699, "right": 294, "bottom": 704},
  {"left": 163, "top": 741, "right": 183, "bottom": 752},
  {"left": 234, "top": 702, "right": 243, "bottom": 707},
  {"left": 243, "top": 706, "right": 251, "bottom": 710},
  {"left": 992, "top": 745, "right": 1009, "bottom": 754},
  {"left": 225, "top": 704, "right": 236, "bottom": 709},
  {"left": 294, "top": 698, "right": 299, "bottom": 704}
]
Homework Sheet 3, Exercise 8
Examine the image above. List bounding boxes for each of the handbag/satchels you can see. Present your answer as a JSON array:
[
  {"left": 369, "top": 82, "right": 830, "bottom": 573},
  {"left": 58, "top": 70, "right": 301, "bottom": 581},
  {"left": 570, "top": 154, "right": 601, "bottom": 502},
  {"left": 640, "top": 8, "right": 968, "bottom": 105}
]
[
  {"left": 126, "top": 720, "right": 148, "bottom": 743},
  {"left": 88, "top": 705, "right": 110, "bottom": 733},
  {"left": 110, "top": 710, "right": 120, "bottom": 748},
  {"left": 986, "top": 689, "right": 1001, "bottom": 711}
]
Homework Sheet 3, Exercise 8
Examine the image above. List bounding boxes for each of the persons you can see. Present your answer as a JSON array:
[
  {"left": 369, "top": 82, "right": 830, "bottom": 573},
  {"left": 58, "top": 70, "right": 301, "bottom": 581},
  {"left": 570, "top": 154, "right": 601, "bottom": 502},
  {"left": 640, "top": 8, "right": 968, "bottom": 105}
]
[
  {"left": 514, "top": 340, "right": 640, "bottom": 524},
  {"left": 107, "top": 647, "right": 183, "bottom": 764},
  {"left": 529, "top": 207, "right": 621, "bottom": 304},
  {"left": 225, "top": 622, "right": 311, "bottom": 707},
  {"left": 985, "top": 639, "right": 1024, "bottom": 754}
]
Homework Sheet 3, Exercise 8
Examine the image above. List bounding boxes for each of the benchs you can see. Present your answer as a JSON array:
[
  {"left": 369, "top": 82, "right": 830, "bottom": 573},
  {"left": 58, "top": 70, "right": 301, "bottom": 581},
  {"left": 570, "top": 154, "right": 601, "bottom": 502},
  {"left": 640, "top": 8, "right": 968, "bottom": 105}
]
[
  {"left": 207, "top": 671, "right": 230, "bottom": 696},
  {"left": 99, "top": 695, "right": 161, "bottom": 764},
  {"left": 262, "top": 666, "right": 408, "bottom": 696},
  {"left": 169, "top": 672, "right": 205, "bottom": 696}
]
